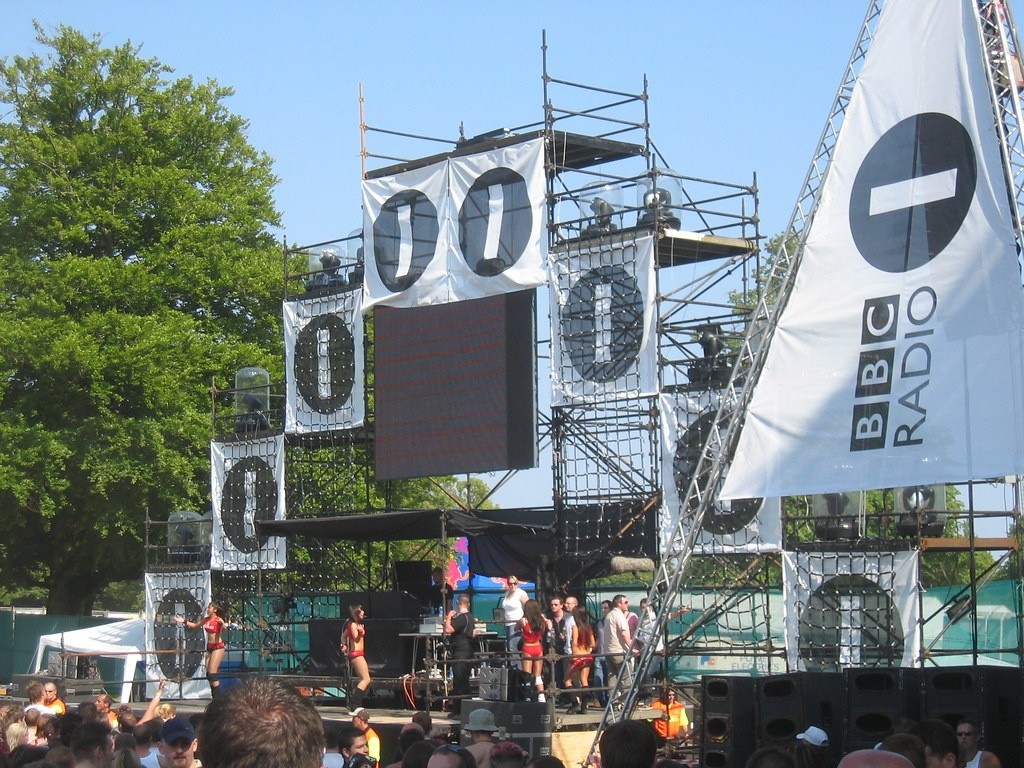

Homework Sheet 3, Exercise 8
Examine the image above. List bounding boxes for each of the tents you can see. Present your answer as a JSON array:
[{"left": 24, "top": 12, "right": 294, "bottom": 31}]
[
  {"left": 35, "top": 616, "right": 146, "bottom": 703},
  {"left": 431, "top": 535, "right": 537, "bottom": 638}
]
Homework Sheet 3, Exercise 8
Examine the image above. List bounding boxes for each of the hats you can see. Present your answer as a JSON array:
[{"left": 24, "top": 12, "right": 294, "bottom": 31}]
[
  {"left": 348, "top": 707, "right": 370, "bottom": 720},
  {"left": 160, "top": 718, "right": 195, "bottom": 743},
  {"left": 464, "top": 709, "right": 500, "bottom": 733},
  {"left": 796, "top": 726, "right": 828, "bottom": 746}
]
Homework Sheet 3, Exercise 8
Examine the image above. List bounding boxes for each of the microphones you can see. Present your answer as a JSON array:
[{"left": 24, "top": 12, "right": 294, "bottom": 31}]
[{"left": 611, "top": 557, "right": 654, "bottom": 573}]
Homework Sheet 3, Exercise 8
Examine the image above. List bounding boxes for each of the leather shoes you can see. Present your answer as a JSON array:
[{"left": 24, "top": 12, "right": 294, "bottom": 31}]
[
  {"left": 566, "top": 703, "right": 581, "bottom": 714},
  {"left": 576, "top": 705, "right": 587, "bottom": 714}
]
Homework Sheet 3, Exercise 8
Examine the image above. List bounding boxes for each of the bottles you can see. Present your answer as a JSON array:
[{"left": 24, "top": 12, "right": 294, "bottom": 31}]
[
  {"left": 439, "top": 605, "right": 444, "bottom": 616},
  {"left": 547, "top": 631, "right": 551, "bottom": 643},
  {"left": 477, "top": 668, "right": 480, "bottom": 675},
  {"left": 471, "top": 668, "right": 475, "bottom": 677},
  {"left": 431, "top": 607, "right": 434, "bottom": 615},
  {"left": 435, "top": 608, "right": 438, "bottom": 614},
  {"left": 448, "top": 666, "right": 453, "bottom": 678},
  {"left": 8, "top": 682, "right": 13, "bottom": 696},
  {"left": 562, "top": 627, "right": 567, "bottom": 637}
]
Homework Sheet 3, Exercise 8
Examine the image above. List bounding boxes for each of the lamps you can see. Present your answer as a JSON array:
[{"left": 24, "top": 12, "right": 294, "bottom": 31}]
[
  {"left": 348, "top": 245, "right": 366, "bottom": 287},
  {"left": 311, "top": 248, "right": 344, "bottom": 291},
  {"left": 638, "top": 188, "right": 680, "bottom": 226},
  {"left": 896, "top": 485, "right": 944, "bottom": 536},
  {"left": 174, "top": 522, "right": 196, "bottom": 551},
  {"left": 816, "top": 493, "right": 859, "bottom": 540},
  {"left": 697, "top": 330, "right": 728, "bottom": 376},
  {"left": 590, "top": 196, "right": 615, "bottom": 231},
  {"left": 236, "top": 392, "right": 267, "bottom": 424}
]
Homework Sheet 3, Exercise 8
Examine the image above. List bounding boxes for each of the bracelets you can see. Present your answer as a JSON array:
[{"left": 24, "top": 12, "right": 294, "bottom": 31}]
[
  {"left": 184, "top": 620, "right": 187, "bottom": 625},
  {"left": 340, "top": 644, "right": 347, "bottom": 647}
]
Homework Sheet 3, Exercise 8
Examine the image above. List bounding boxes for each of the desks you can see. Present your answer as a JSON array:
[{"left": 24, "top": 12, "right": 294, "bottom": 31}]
[{"left": 398, "top": 631, "right": 498, "bottom": 677}]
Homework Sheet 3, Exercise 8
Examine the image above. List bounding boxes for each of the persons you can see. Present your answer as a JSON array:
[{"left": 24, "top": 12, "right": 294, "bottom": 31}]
[
  {"left": 173, "top": 602, "right": 240, "bottom": 698},
  {"left": 512, "top": 599, "right": 547, "bottom": 703},
  {"left": 1, "top": 678, "right": 383, "bottom": 768},
  {"left": 545, "top": 594, "right": 692, "bottom": 715},
  {"left": 501, "top": 574, "right": 529, "bottom": 670},
  {"left": 591, "top": 718, "right": 660, "bottom": 768},
  {"left": 744, "top": 719, "right": 1003, "bottom": 768},
  {"left": 443, "top": 595, "right": 478, "bottom": 721},
  {"left": 386, "top": 710, "right": 567, "bottom": 768},
  {"left": 339, "top": 603, "right": 370, "bottom": 713},
  {"left": 641, "top": 687, "right": 690, "bottom": 750}
]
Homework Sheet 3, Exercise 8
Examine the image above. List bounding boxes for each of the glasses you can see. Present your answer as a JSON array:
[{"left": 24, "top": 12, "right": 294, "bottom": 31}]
[
  {"left": 621, "top": 601, "right": 628, "bottom": 605},
  {"left": 508, "top": 583, "right": 518, "bottom": 585},
  {"left": 550, "top": 604, "right": 561, "bottom": 606},
  {"left": 436, "top": 743, "right": 465, "bottom": 754},
  {"left": 668, "top": 694, "right": 675, "bottom": 696},
  {"left": 564, "top": 603, "right": 576, "bottom": 605},
  {"left": 957, "top": 731, "right": 976, "bottom": 736},
  {"left": 46, "top": 690, "right": 56, "bottom": 693}
]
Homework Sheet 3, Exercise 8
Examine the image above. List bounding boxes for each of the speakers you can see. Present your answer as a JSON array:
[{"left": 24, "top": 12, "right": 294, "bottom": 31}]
[{"left": 479, "top": 668, "right": 538, "bottom": 702}]
